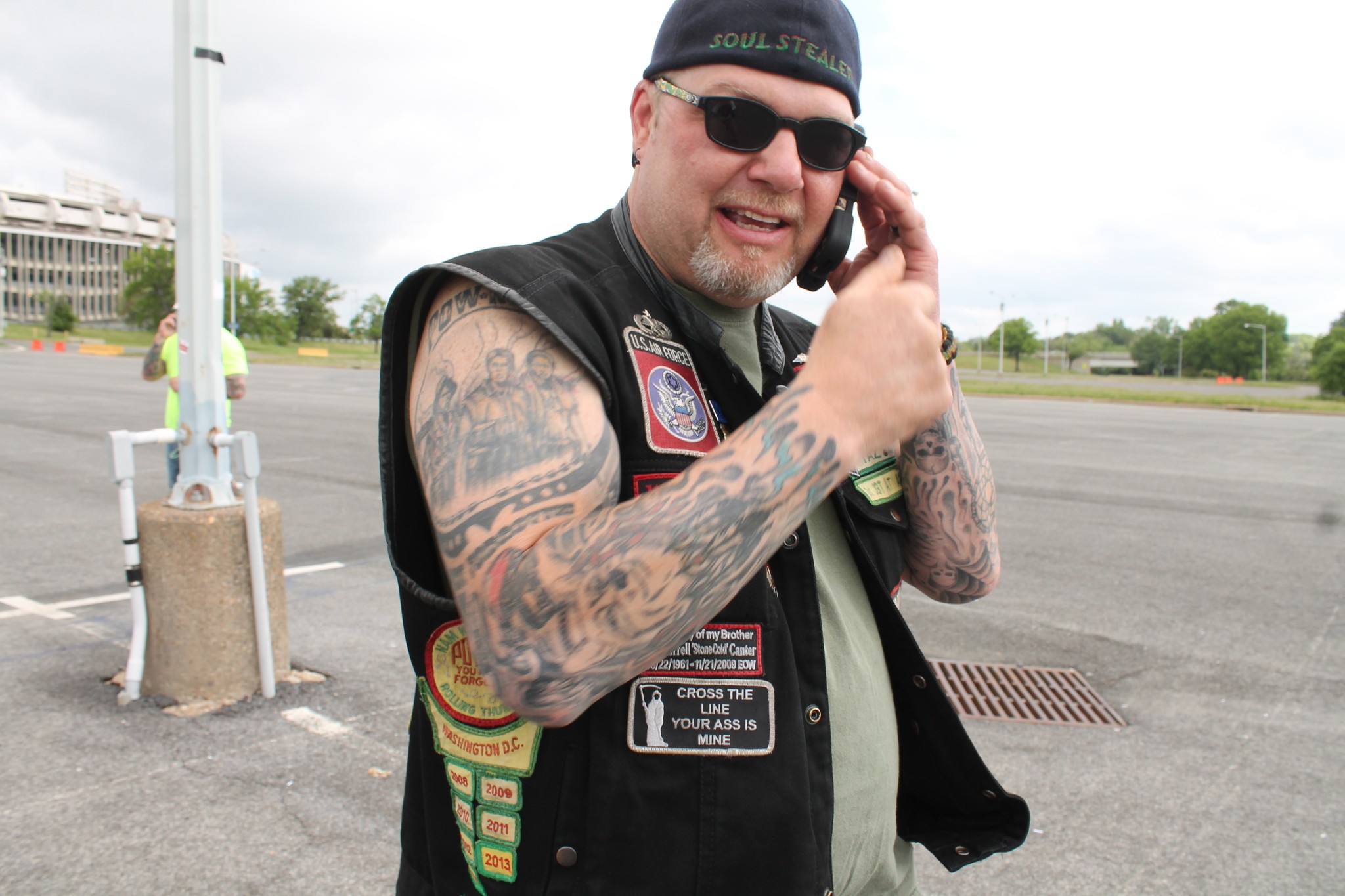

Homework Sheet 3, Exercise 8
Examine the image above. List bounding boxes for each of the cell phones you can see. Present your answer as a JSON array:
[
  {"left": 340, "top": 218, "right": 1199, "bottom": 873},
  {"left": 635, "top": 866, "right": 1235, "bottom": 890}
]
[{"left": 797, "top": 124, "right": 865, "bottom": 292}]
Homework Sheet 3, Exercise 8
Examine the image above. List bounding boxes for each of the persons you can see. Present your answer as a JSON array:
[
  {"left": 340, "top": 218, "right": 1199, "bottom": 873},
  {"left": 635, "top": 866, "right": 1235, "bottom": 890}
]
[
  {"left": 141, "top": 299, "right": 249, "bottom": 496},
  {"left": 377, "top": 0, "right": 1030, "bottom": 896}
]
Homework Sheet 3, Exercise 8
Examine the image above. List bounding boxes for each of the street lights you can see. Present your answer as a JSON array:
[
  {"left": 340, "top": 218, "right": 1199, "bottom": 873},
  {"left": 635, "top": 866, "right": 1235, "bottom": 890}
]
[
  {"left": 1165, "top": 334, "right": 1182, "bottom": 376},
  {"left": 1245, "top": 323, "right": 1266, "bottom": 379}
]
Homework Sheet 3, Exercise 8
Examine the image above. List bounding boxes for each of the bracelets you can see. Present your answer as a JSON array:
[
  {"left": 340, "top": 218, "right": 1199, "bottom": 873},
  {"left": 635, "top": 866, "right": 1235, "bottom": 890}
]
[{"left": 934, "top": 322, "right": 959, "bottom": 366}]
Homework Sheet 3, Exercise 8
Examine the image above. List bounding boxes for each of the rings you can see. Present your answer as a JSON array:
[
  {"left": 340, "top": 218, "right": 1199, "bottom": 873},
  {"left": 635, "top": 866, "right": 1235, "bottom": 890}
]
[{"left": 889, "top": 226, "right": 899, "bottom": 233}]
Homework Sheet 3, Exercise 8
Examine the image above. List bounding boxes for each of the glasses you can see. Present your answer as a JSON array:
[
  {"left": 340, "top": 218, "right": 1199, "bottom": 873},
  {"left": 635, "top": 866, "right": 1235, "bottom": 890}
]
[{"left": 653, "top": 78, "right": 868, "bottom": 172}]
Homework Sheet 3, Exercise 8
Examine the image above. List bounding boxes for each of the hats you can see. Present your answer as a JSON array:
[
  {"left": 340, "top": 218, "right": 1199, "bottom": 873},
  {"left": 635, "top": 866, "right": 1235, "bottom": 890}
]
[{"left": 641, "top": 0, "right": 862, "bottom": 119}]
